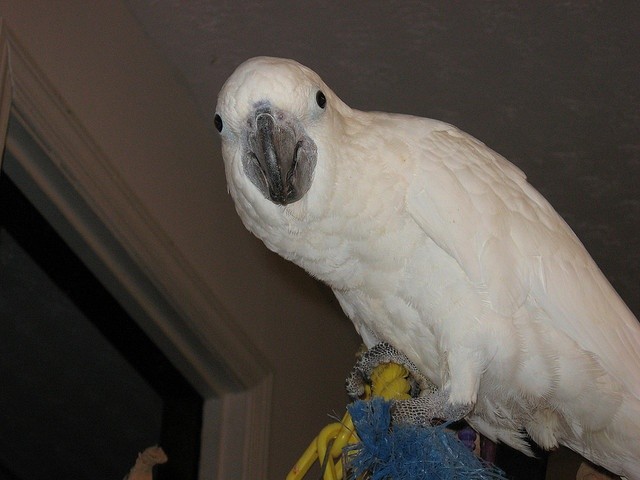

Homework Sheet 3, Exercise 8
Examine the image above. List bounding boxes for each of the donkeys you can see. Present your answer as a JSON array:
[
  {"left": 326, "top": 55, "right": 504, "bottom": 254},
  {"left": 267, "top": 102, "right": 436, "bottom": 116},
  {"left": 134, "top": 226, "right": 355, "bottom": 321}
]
[{"left": 213, "top": 56, "right": 640, "bottom": 478}]
